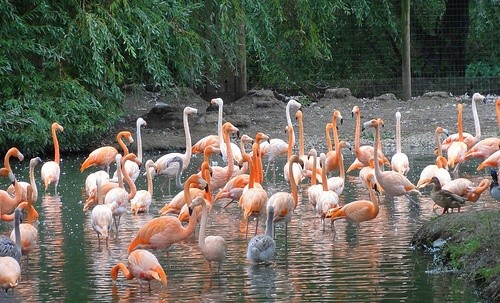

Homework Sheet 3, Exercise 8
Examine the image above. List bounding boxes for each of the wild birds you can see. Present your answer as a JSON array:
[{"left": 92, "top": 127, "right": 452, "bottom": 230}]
[{"left": 0, "top": 87, "right": 500, "bottom": 303}]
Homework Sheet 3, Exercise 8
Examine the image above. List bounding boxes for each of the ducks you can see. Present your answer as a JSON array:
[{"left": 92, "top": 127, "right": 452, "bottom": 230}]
[
  {"left": 487, "top": 166, "right": 500, "bottom": 201},
  {"left": 427, "top": 176, "right": 469, "bottom": 215}
]
